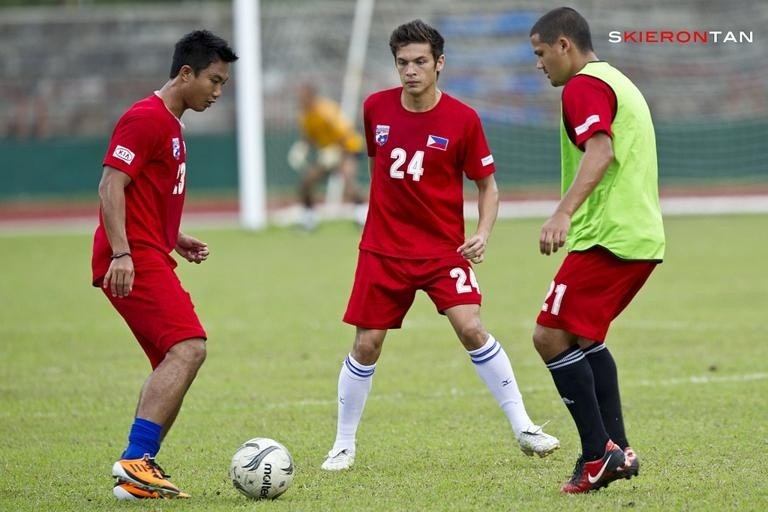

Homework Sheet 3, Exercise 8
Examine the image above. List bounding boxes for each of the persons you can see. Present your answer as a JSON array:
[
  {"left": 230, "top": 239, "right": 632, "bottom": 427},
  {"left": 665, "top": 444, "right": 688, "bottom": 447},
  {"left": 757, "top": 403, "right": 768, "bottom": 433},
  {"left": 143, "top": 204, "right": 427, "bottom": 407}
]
[
  {"left": 92, "top": 29, "right": 239, "bottom": 500},
  {"left": 530, "top": 8, "right": 665, "bottom": 494},
  {"left": 321, "top": 19, "right": 560, "bottom": 471},
  {"left": 287, "top": 78, "right": 368, "bottom": 226}
]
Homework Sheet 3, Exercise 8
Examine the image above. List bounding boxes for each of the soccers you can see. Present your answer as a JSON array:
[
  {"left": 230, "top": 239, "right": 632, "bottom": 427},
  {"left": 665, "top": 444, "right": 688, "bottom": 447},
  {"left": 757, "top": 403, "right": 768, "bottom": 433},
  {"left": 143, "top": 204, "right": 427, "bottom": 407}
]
[{"left": 230, "top": 437, "right": 294, "bottom": 499}]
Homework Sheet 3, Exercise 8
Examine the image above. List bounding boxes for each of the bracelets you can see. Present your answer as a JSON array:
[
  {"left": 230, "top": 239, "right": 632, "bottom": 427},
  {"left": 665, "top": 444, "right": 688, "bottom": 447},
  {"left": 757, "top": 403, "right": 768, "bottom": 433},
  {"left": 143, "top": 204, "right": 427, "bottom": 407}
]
[{"left": 111, "top": 252, "right": 132, "bottom": 260}]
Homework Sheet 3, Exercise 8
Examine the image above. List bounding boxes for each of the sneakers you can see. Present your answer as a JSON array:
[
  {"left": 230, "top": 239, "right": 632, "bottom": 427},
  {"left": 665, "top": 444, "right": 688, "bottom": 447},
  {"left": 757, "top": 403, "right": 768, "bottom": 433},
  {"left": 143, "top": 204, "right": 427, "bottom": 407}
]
[
  {"left": 112, "top": 453, "right": 193, "bottom": 500},
  {"left": 518, "top": 424, "right": 561, "bottom": 458},
  {"left": 320, "top": 447, "right": 355, "bottom": 472},
  {"left": 559, "top": 438, "right": 640, "bottom": 495}
]
